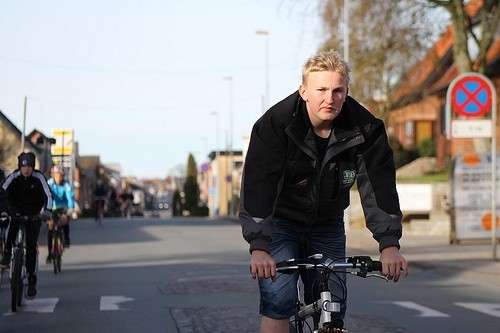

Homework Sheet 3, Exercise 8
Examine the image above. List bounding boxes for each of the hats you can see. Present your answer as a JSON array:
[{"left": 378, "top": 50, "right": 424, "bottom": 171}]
[{"left": 18, "top": 152, "right": 35, "bottom": 170}]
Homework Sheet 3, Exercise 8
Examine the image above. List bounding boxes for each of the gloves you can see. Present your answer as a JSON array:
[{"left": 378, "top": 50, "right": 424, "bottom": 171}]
[
  {"left": 0, "top": 215, "right": 9, "bottom": 230},
  {"left": 39, "top": 210, "right": 51, "bottom": 223}
]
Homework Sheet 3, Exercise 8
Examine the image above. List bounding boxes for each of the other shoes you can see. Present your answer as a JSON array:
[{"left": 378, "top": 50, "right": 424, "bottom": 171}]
[
  {"left": 0, "top": 253, "right": 12, "bottom": 269},
  {"left": 26, "top": 277, "right": 37, "bottom": 299}
]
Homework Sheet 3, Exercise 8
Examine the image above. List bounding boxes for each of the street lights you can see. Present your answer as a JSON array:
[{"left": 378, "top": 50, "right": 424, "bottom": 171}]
[
  {"left": 223, "top": 76, "right": 234, "bottom": 172},
  {"left": 256, "top": 29, "right": 269, "bottom": 109}
]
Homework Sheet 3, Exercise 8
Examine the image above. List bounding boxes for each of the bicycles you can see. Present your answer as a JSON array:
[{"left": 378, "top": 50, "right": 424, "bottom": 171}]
[
  {"left": 0, "top": 209, "right": 65, "bottom": 312},
  {"left": 250, "top": 254, "right": 403, "bottom": 333}
]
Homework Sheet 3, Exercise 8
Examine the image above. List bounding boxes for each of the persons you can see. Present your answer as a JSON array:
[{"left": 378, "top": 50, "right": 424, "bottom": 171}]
[
  {"left": 46, "top": 165, "right": 75, "bottom": 263},
  {"left": 93, "top": 179, "right": 108, "bottom": 222},
  {"left": 0, "top": 152, "right": 54, "bottom": 299},
  {"left": 237, "top": 50, "right": 409, "bottom": 333},
  {"left": 120, "top": 190, "right": 134, "bottom": 217}
]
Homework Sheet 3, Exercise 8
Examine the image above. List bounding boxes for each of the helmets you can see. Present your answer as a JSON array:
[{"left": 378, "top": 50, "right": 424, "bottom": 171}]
[{"left": 51, "top": 165, "right": 65, "bottom": 176}]
